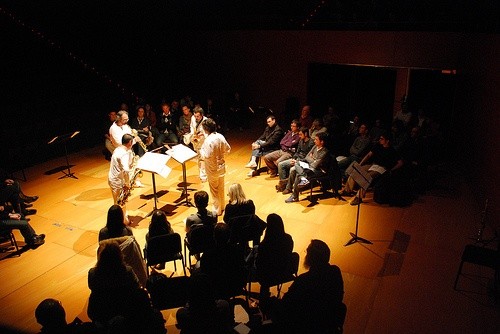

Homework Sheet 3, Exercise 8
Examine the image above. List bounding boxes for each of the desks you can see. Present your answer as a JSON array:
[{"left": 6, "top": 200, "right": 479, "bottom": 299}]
[{"left": 452, "top": 239, "right": 498, "bottom": 291}]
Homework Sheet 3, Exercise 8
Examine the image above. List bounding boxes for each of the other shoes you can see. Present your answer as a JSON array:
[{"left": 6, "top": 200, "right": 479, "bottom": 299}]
[
  {"left": 27, "top": 233, "right": 45, "bottom": 249},
  {"left": 25, "top": 196, "right": 39, "bottom": 203},
  {"left": 25, "top": 209, "right": 37, "bottom": 216},
  {"left": 350, "top": 196, "right": 363, "bottom": 205},
  {"left": 245, "top": 161, "right": 257, "bottom": 168},
  {"left": 270, "top": 173, "right": 276, "bottom": 177},
  {"left": 296, "top": 179, "right": 310, "bottom": 188},
  {"left": 285, "top": 195, "right": 299, "bottom": 203},
  {"left": 282, "top": 189, "right": 292, "bottom": 194},
  {"left": 339, "top": 190, "right": 354, "bottom": 196}
]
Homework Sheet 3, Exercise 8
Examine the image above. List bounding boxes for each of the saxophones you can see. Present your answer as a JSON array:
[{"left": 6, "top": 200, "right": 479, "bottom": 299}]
[{"left": 116, "top": 168, "right": 143, "bottom": 206}]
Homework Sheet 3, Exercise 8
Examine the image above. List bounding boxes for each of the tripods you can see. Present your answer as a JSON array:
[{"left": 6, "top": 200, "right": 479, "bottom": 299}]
[
  {"left": 147, "top": 163, "right": 196, "bottom": 217},
  {"left": 344, "top": 165, "right": 374, "bottom": 246},
  {"left": 49, "top": 132, "right": 78, "bottom": 180}
]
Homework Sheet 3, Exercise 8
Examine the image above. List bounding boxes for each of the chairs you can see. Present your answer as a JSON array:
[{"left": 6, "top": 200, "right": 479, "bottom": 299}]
[
  {"left": 0, "top": 233, "right": 21, "bottom": 257},
  {"left": 97, "top": 214, "right": 347, "bottom": 334},
  {"left": 258, "top": 145, "right": 340, "bottom": 203}
]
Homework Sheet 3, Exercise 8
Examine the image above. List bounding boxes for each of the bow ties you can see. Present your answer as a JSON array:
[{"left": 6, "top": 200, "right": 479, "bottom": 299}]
[{"left": 162, "top": 114, "right": 171, "bottom": 120}]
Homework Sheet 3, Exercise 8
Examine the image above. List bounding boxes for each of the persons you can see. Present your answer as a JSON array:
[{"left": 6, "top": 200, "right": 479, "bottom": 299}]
[
  {"left": 178, "top": 182, "right": 345, "bottom": 334},
  {"left": 87, "top": 244, "right": 139, "bottom": 321},
  {"left": 186, "top": 105, "right": 211, "bottom": 183},
  {"left": 35, "top": 299, "right": 93, "bottom": 334},
  {"left": 109, "top": 134, "right": 136, "bottom": 226},
  {"left": 98, "top": 205, "right": 133, "bottom": 242},
  {"left": 248, "top": 102, "right": 462, "bottom": 206},
  {"left": 106, "top": 98, "right": 194, "bottom": 156},
  {"left": 200, "top": 118, "right": 231, "bottom": 216},
  {"left": 144, "top": 209, "right": 174, "bottom": 271},
  {"left": 0, "top": 176, "right": 46, "bottom": 248}
]
